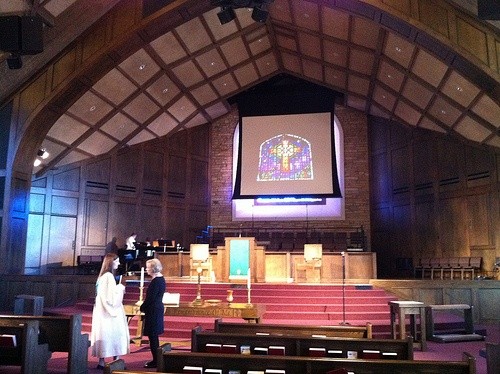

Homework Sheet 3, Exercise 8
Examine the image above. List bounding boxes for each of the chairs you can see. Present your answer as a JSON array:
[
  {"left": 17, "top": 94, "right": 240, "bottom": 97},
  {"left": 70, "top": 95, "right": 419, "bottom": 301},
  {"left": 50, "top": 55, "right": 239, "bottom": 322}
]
[
  {"left": 422, "top": 258, "right": 440, "bottom": 281},
  {"left": 451, "top": 257, "right": 471, "bottom": 280},
  {"left": 414, "top": 258, "right": 431, "bottom": 280},
  {"left": 441, "top": 257, "right": 460, "bottom": 281},
  {"left": 432, "top": 257, "right": 449, "bottom": 280},
  {"left": 76, "top": 254, "right": 106, "bottom": 275},
  {"left": 211, "top": 230, "right": 364, "bottom": 284},
  {"left": 461, "top": 256, "right": 483, "bottom": 280}
]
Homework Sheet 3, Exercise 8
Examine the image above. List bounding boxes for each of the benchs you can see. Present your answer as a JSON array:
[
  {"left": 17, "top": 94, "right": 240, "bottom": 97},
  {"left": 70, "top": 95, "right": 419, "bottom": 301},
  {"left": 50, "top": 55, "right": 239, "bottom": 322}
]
[{"left": 0, "top": 314, "right": 500, "bottom": 374}]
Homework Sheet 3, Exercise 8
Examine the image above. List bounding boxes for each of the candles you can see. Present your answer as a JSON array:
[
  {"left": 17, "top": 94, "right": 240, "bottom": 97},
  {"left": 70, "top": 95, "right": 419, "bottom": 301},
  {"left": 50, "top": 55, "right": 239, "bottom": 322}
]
[
  {"left": 140, "top": 267, "right": 145, "bottom": 288},
  {"left": 247, "top": 268, "right": 251, "bottom": 289}
]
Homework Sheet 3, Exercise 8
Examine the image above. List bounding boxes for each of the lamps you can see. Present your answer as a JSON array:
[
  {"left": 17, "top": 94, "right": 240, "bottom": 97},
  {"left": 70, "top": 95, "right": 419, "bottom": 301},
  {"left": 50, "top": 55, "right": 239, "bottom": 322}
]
[
  {"left": 250, "top": 3, "right": 270, "bottom": 24},
  {"left": 37, "top": 149, "right": 49, "bottom": 160},
  {"left": 217, "top": 5, "right": 237, "bottom": 26}
]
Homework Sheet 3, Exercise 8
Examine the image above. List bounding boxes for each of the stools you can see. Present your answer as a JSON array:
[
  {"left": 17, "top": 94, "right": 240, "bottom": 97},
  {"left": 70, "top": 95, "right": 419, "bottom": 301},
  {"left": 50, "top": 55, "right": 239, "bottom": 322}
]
[
  {"left": 388, "top": 300, "right": 428, "bottom": 352},
  {"left": 12, "top": 293, "right": 45, "bottom": 316}
]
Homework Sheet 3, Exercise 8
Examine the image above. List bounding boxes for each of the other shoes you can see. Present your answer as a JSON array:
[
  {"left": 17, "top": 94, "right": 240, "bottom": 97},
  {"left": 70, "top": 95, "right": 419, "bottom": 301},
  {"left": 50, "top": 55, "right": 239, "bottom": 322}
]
[
  {"left": 144, "top": 360, "right": 158, "bottom": 368},
  {"left": 97, "top": 364, "right": 105, "bottom": 370}
]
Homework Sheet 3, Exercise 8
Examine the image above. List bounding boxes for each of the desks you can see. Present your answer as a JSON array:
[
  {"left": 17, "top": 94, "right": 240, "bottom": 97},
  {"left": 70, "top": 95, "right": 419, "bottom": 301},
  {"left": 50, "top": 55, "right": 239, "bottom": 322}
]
[{"left": 136, "top": 300, "right": 266, "bottom": 348}]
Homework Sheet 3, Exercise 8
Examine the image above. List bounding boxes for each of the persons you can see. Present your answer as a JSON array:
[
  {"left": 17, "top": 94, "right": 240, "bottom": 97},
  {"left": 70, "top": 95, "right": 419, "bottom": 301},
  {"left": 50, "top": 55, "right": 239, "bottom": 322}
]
[
  {"left": 126, "top": 233, "right": 136, "bottom": 271},
  {"left": 140, "top": 259, "right": 166, "bottom": 369},
  {"left": 91, "top": 253, "right": 130, "bottom": 369}
]
